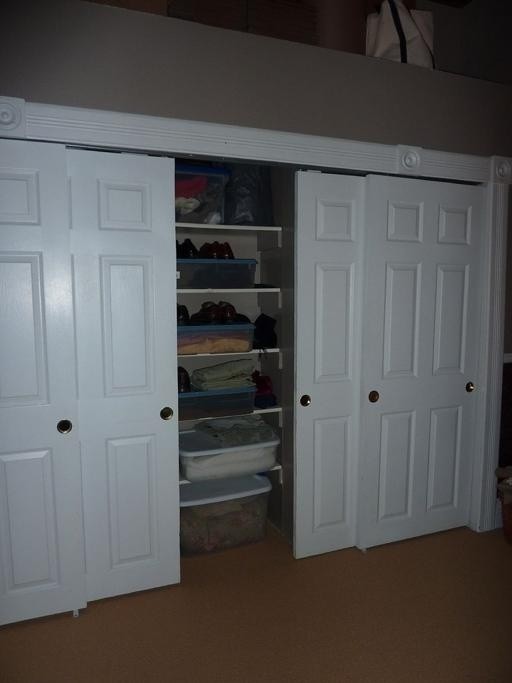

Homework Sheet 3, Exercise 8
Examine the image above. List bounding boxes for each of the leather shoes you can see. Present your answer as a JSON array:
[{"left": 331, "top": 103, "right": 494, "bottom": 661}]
[
  {"left": 178, "top": 301, "right": 237, "bottom": 326},
  {"left": 175, "top": 239, "right": 235, "bottom": 259},
  {"left": 178, "top": 366, "right": 190, "bottom": 392}
]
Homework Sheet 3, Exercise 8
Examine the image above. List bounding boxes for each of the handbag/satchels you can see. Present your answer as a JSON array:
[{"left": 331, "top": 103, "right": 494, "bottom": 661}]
[{"left": 366, "top": 0, "right": 436, "bottom": 72}]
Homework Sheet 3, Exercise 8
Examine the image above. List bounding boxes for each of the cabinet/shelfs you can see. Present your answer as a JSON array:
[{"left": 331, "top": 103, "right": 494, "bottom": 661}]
[{"left": 1, "top": 96, "right": 511, "bottom": 626}]
